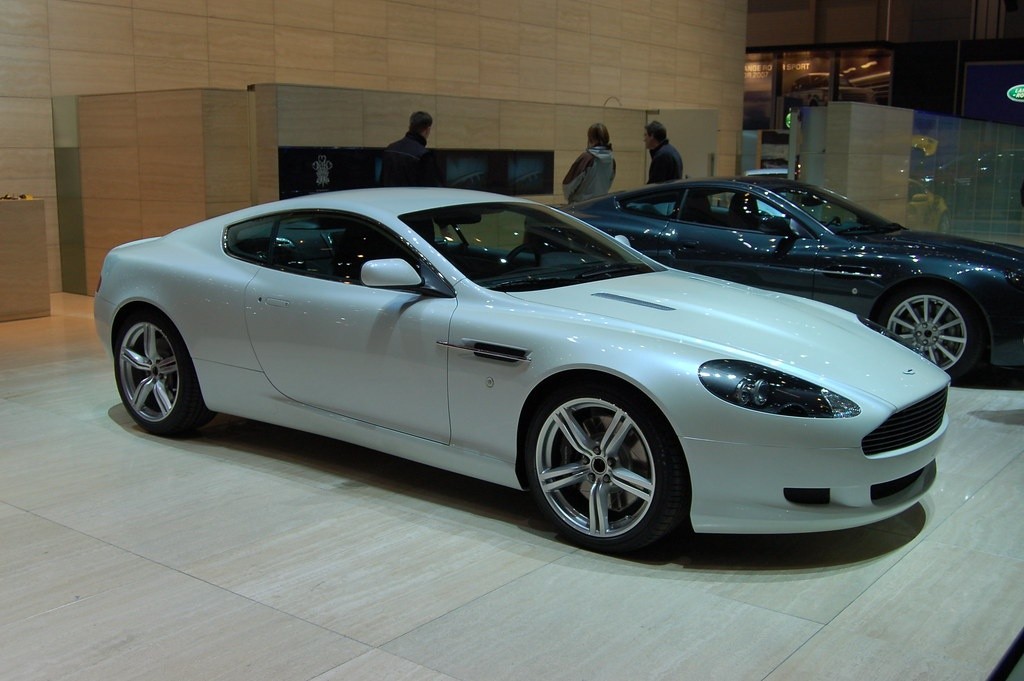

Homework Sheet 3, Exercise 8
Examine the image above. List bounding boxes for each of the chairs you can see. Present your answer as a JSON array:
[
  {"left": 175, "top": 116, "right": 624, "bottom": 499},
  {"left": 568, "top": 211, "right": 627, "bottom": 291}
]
[
  {"left": 727, "top": 193, "right": 761, "bottom": 231},
  {"left": 675, "top": 191, "right": 715, "bottom": 226}
]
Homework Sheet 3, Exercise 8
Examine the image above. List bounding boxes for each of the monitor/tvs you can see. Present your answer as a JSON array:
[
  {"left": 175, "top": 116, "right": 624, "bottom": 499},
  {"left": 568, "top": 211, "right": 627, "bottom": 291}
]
[{"left": 279, "top": 145, "right": 555, "bottom": 200}]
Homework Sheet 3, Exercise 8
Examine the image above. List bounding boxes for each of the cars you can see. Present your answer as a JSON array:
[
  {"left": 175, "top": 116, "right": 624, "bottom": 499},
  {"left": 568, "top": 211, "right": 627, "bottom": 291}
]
[
  {"left": 95, "top": 186, "right": 952, "bottom": 556},
  {"left": 521, "top": 177, "right": 1024, "bottom": 384},
  {"left": 742, "top": 169, "right": 793, "bottom": 218}
]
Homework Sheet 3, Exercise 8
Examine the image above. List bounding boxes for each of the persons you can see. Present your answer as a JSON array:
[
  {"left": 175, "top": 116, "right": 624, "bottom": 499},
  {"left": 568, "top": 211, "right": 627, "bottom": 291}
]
[
  {"left": 643, "top": 121, "right": 683, "bottom": 183},
  {"left": 562, "top": 123, "right": 617, "bottom": 204},
  {"left": 378, "top": 111, "right": 453, "bottom": 241}
]
